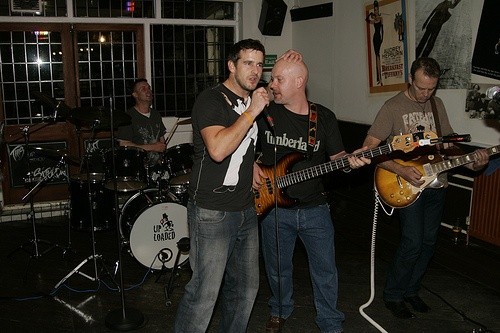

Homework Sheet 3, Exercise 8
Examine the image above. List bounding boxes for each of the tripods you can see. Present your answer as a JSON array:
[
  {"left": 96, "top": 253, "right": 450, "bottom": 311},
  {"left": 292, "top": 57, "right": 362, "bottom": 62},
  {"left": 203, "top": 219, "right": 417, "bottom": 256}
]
[{"left": 7, "top": 114, "right": 121, "bottom": 294}]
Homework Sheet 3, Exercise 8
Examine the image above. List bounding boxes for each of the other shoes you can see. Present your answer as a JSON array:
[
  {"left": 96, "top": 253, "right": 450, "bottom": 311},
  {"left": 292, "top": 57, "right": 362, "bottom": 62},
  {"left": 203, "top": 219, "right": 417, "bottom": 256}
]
[
  {"left": 266, "top": 315, "right": 285, "bottom": 331},
  {"left": 335, "top": 327, "right": 344, "bottom": 333},
  {"left": 153, "top": 284, "right": 166, "bottom": 303},
  {"left": 139, "top": 283, "right": 155, "bottom": 299}
]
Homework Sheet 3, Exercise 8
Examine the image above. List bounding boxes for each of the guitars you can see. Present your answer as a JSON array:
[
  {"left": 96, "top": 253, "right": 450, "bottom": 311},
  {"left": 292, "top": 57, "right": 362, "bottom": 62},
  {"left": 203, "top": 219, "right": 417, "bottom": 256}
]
[
  {"left": 374, "top": 144, "right": 500, "bottom": 208},
  {"left": 254, "top": 125, "right": 441, "bottom": 219}
]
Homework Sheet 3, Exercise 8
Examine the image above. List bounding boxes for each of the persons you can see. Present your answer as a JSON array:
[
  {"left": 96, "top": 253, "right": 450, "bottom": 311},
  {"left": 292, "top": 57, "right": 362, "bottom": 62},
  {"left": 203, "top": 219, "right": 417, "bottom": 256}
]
[
  {"left": 360, "top": 57, "right": 489, "bottom": 320},
  {"left": 251, "top": 54, "right": 371, "bottom": 333},
  {"left": 115, "top": 78, "right": 170, "bottom": 158},
  {"left": 173, "top": 38, "right": 303, "bottom": 333}
]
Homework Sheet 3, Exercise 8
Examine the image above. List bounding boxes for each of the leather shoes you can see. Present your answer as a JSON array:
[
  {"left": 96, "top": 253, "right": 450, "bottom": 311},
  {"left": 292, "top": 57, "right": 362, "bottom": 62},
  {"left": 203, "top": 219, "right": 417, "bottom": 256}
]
[
  {"left": 402, "top": 295, "right": 431, "bottom": 312},
  {"left": 385, "top": 300, "right": 416, "bottom": 320}
]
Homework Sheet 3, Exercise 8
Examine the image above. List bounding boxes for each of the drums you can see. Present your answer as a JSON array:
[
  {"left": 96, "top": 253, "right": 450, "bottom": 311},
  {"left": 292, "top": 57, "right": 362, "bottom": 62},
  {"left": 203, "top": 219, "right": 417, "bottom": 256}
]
[
  {"left": 104, "top": 145, "right": 149, "bottom": 191},
  {"left": 159, "top": 143, "right": 193, "bottom": 183},
  {"left": 69, "top": 172, "right": 116, "bottom": 232},
  {"left": 118, "top": 188, "right": 189, "bottom": 272}
]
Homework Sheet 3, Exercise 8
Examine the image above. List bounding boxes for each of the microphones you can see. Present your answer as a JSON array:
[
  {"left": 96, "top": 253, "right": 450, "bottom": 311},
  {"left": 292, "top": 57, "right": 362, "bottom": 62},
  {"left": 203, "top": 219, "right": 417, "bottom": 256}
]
[{"left": 262, "top": 104, "right": 277, "bottom": 131}]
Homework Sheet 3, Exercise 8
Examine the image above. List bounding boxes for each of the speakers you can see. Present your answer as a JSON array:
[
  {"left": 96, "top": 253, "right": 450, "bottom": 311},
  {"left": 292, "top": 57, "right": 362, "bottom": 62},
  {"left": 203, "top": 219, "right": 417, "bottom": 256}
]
[{"left": 257, "top": 0, "right": 288, "bottom": 37}]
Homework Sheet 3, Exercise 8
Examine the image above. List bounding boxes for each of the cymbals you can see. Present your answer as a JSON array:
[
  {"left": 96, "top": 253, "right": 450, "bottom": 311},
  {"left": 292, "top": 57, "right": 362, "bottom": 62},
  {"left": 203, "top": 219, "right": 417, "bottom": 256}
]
[
  {"left": 29, "top": 89, "right": 71, "bottom": 118},
  {"left": 64, "top": 106, "right": 132, "bottom": 127},
  {"left": 176, "top": 118, "right": 193, "bottom": 125}
]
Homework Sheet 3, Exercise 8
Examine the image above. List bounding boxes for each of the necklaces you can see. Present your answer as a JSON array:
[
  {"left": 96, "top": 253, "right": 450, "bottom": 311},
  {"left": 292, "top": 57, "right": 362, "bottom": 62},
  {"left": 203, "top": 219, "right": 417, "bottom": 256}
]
[{"left": 408, "top": 91, "right": 427, "bottom": 117}]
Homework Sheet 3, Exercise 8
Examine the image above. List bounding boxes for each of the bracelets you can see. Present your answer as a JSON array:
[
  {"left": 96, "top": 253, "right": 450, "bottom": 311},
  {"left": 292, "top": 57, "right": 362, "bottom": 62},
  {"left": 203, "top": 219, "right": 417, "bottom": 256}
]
[{"left": 243, "top": 112, "right": 253, "bottom": 125}]
